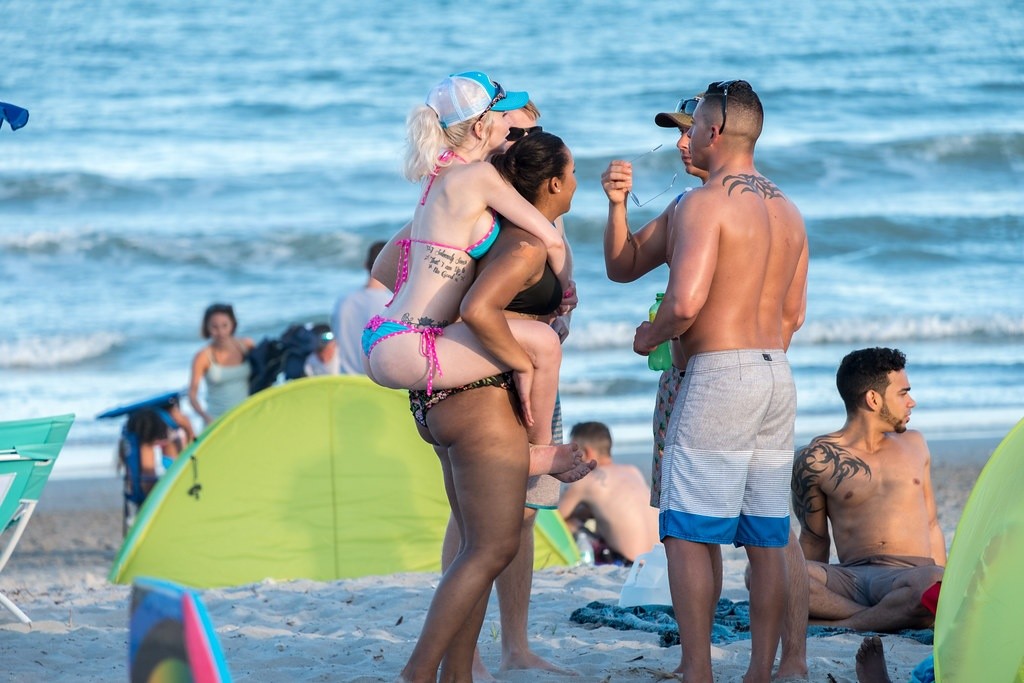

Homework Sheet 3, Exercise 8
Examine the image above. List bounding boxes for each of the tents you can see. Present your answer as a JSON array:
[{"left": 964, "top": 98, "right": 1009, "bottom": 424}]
[
  {"left": 110, "top": 374, "right": 581, "bottom": 587},
  {"left": 933, "top": 416, "right": 1024, "bottom": 683}
]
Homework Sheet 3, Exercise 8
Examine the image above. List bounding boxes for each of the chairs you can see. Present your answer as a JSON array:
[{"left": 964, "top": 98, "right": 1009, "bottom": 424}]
[
  {"left": 118, "top": 424, "right": 158, "bottom": 539},
  {"left": 0, "top": 414, "right": 76, "bottom": 626}
]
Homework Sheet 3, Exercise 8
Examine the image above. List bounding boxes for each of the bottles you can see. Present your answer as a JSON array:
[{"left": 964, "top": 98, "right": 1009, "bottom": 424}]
[{"left": 648, "top": 292, "right": 675, "bottom": 371}]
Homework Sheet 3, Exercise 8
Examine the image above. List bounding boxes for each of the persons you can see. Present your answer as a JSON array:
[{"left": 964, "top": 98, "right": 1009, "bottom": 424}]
[
  {"left": 303, "top": 240, "right": 396, "bottom": 376},
  {"left": 601, "top": 80, "right": 809, "bottom": 683},
  {"left": 360, "top": 69, "right": 578, "bottom": 683},
  {"left": 856, "top": 635, "right": 893, "bottom": 683},
  {"left": 558, "top": 421, "right": 666, "bottom": 568},
  {"left": 118, "top": 302, "right": 257, "bottom": 506},
  {"left": 745, "top": 346, "right": 947, "bottom": 632}
]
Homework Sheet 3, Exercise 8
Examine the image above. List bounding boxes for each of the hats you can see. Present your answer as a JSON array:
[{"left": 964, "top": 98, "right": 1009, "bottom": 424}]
[
  {"left": 425, "top": 70, "right": 529, "bottom": 128},
  {"left": 654, "top": 112, "right": 692, "bottom": 129}
]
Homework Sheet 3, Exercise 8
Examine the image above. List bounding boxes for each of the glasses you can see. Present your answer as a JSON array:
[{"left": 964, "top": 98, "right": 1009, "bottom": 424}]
[
  {"left": 717, "top": 79, "right": 752, "bottom": 136},
  {"left": 623, "top": 144, "right": 677, "bottom": 207},
  {"left": 506, "top": 126, "right": 542, "bottom": 141},
  {"left": 472, "top": 81, "right": 507, "bottom": 131},
  {"left": 675, "top": 99, "right": 698, "bottom": 116}
]
